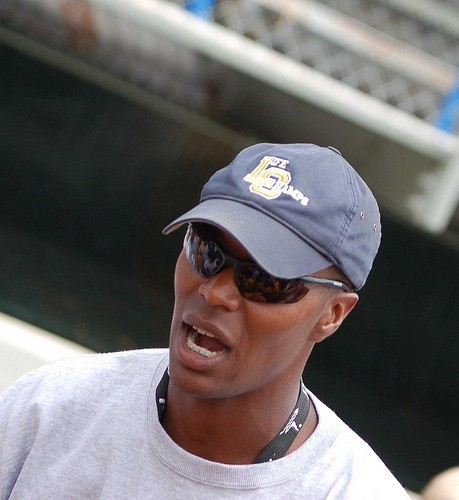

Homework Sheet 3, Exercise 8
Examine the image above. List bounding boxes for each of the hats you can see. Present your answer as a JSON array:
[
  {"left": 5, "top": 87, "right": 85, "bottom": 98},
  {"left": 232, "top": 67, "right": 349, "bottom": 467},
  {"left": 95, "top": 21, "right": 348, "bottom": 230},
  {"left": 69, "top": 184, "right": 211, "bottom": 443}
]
[{"left": 162, "top": 142, "right": 382, "bottom": 290}]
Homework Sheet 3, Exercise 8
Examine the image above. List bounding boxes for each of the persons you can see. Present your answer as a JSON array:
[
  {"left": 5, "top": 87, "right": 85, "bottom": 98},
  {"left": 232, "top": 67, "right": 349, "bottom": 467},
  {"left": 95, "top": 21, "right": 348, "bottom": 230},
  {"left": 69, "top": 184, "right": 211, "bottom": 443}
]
[{"left": 1, "top": 141, "right": 413, "bottom": 499}]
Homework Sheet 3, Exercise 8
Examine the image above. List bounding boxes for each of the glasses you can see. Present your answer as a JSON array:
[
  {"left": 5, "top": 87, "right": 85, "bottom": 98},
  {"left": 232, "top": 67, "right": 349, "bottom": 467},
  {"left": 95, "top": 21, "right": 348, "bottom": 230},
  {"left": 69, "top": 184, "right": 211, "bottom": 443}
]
[{"left": 182, "top": 221, "right": 354, "bottom": 304}]
